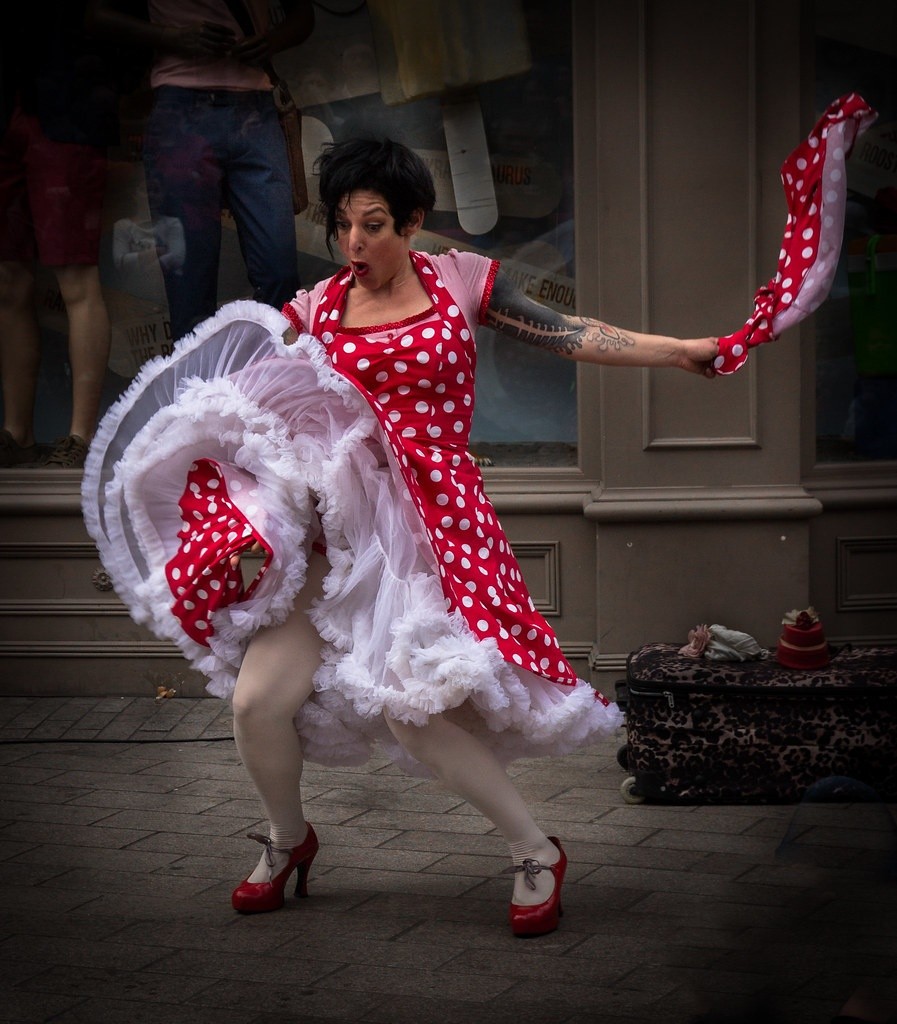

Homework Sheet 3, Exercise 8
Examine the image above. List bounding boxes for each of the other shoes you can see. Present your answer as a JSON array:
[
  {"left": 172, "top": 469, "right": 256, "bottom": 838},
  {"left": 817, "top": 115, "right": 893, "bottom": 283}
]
[
  {"left": 46, "top": 435, "right": 89, "bottom": 468},
  {"left": 0, "top": 429, "right": 40, "bottom": 468}
]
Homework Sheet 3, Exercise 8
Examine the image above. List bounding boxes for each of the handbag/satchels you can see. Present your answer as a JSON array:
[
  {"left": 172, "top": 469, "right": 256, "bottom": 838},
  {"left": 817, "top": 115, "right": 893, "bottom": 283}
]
[{"left": 220, "top": 85, "right": 309, "bottom": 215}]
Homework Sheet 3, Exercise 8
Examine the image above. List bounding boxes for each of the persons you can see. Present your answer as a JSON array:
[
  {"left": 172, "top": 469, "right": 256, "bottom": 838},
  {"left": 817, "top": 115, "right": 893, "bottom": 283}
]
[
  {"left": 81, "top": 137, "right": 722, "bottom": 937},
  {"left": 0, "top": 0, "right": 122, "bottom": 471},
  {"left": 102, "top": 0, "right": 307, "bottom": 352}
]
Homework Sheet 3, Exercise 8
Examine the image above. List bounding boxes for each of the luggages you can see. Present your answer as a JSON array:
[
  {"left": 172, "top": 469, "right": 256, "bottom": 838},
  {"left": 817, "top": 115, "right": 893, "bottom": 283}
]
[{"left": 614, "top": 642, "right": 897, "bottom": 805}]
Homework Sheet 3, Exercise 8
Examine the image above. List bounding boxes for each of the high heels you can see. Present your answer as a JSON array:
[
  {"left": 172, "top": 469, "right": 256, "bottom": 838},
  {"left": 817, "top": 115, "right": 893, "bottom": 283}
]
[
  {"left": 508, "top": 836, "right": 567, "bottom": 935},
  {"left": 232, "top": 820, "right": 319, "bottom": 912}
]
